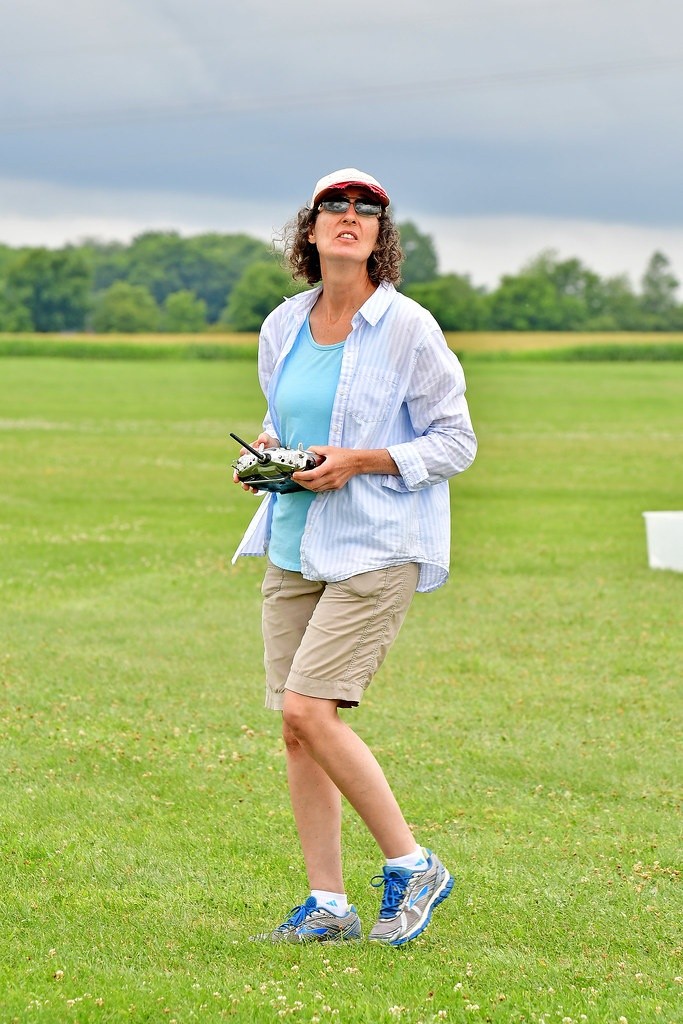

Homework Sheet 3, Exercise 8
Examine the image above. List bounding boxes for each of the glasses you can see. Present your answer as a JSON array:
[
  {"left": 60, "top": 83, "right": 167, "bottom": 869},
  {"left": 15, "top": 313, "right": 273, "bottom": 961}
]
[{"left": 315, "top": 193, "right": 384, "bottom": 221}]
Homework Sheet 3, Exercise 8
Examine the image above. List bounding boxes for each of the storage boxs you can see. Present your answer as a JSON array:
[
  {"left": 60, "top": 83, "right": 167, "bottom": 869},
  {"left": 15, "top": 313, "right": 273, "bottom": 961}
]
[{"left": 641, "top": 510, "right": 683, "bottom": 575}]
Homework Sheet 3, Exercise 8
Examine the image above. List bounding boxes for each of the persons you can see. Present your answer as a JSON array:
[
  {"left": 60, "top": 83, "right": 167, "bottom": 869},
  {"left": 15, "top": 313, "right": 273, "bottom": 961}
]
[{"left": 231, "top": 166, "right": 481, "bottom": 945}]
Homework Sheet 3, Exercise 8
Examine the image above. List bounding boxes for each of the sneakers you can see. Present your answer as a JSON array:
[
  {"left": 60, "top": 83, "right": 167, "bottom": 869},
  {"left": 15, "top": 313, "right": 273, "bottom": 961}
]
[
  {"left": 246, "top": 897, "right": 360, "bottom": 947},
  {"left": 368, "top": 849, "right": 455, "bottom": 948}
]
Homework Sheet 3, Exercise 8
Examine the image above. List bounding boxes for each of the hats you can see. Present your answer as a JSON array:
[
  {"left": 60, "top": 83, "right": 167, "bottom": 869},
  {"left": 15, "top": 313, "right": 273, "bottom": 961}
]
[{"left": 308, "top": 168, "right": 389, "bottom": 210}]
